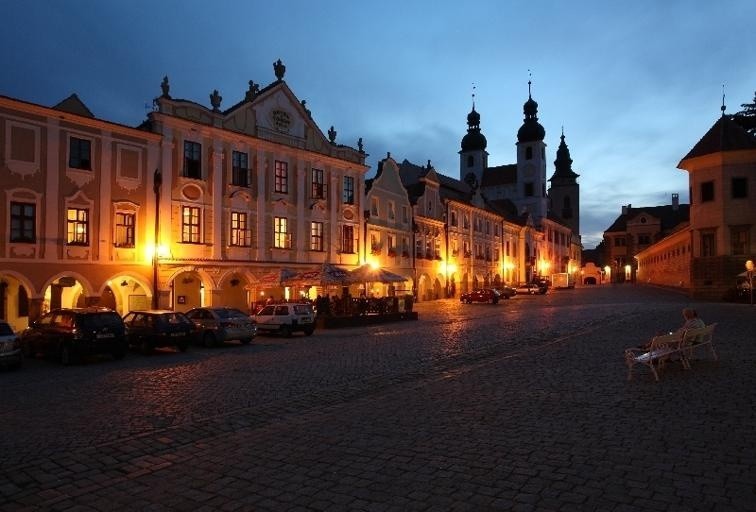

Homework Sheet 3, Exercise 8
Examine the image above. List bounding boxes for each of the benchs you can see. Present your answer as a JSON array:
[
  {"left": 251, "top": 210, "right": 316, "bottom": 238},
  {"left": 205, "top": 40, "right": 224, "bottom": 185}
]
[{"left": 626, "top": 323, "right": 718, "bottom": 382}]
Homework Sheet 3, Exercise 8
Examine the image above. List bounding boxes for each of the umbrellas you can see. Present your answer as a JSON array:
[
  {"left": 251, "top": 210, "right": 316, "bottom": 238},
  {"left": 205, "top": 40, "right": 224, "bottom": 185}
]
[
  {"left": 281, "top": 259, "right": 352, "bottom": 297},
  {"left": 243, "top": 268, "right": 305, "bottom": 291},
  {"left": 341, "top": 263, "right": 408, "bottom": 283}
]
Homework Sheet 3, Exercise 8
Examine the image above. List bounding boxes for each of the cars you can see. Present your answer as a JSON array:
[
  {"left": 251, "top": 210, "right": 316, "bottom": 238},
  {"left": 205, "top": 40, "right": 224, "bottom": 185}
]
[
  {"left": 183, "top": 305, "right": 257, "bottom": 348},
  {"left": 0, "top": 321, "right": 23, "bottom": 372},
  {"left": 460, "top": 281, "right": 547, "bottom": 305}
]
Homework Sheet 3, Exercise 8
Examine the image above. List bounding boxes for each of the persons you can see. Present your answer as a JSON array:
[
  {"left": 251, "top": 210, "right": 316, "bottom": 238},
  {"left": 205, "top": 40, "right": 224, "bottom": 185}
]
[
  {"left": 266, "top": 290, "right": 371, "bottom": 317},
  {"left": 649, "top": 306, "right": 707, "bottom": 363}
]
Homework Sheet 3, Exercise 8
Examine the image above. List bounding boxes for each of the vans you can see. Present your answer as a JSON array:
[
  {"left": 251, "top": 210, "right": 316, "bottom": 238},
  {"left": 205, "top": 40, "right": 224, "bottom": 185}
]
[{"left": 20, "top": 306, "right": 127, "bottom": 366}]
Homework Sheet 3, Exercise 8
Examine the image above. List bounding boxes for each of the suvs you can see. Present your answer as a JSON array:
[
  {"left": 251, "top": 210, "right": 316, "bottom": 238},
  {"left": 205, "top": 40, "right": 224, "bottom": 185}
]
[
  {"left": 122, "top": 309, "right": 193, "bottom": 356},
  {"left": 248, "top": 302, "right": 316, "bottom": 338}
]
